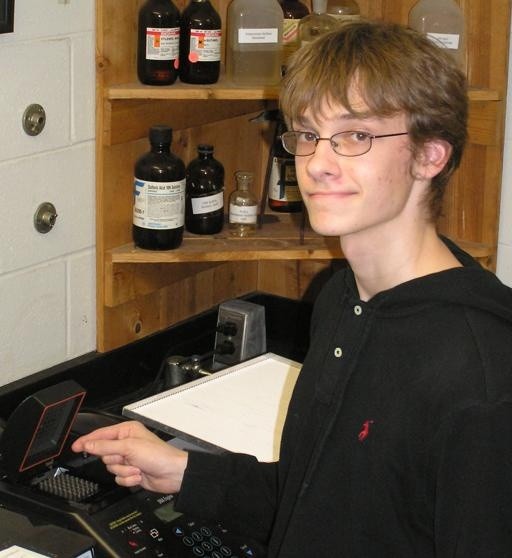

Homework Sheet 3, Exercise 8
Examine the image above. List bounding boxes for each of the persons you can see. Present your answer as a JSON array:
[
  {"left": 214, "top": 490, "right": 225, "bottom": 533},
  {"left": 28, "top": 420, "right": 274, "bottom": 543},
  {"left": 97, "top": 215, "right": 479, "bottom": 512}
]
[{"left": 69, "top": 18, "right": 511, "bottom": 557}]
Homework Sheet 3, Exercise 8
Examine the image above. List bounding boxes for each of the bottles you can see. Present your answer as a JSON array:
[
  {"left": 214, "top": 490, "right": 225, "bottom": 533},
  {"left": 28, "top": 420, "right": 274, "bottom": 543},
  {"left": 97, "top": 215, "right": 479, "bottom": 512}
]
[
  {"left": 278, "top": 1, "right": 311, "bottom": 78},
  {"left": 408, "top": 1, "right": 469, "bottom": 78},
  {"left": 225, "top": 1, "right": 284, "bottom": 85},
  {"left": 225, "top": 168, "right": 263, "bottom": 245},
  {"left": 129, "top": 122, "right": 187, "bottom": 252},
  {"left": 136, "top": 1, "right": 179, "bottom": 88},
  {"left": 326, "top": 1, "right": 361, "bottom": 25},
  {"left": 184, "top": 140, "right": 228, "bottom": 236},
  {"left": 297, "top": 1, "right": 340, "bottom": 48},
  {"left": 178, "top": 1, "right": 222, "bottom": 85}
]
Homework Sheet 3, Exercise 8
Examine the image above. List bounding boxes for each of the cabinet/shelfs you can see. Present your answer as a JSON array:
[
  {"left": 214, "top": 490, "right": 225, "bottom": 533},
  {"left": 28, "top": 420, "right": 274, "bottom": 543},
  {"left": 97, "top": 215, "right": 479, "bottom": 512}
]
[{"left": 95, "top": 2, "right": 510, "bottom": 351}]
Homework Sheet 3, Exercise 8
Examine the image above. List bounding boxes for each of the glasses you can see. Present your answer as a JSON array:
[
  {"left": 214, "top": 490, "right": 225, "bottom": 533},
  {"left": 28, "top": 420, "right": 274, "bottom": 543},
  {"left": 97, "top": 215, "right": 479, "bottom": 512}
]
[{"left": 278, "top": 130, "right": 410, "bottom": 158}]
